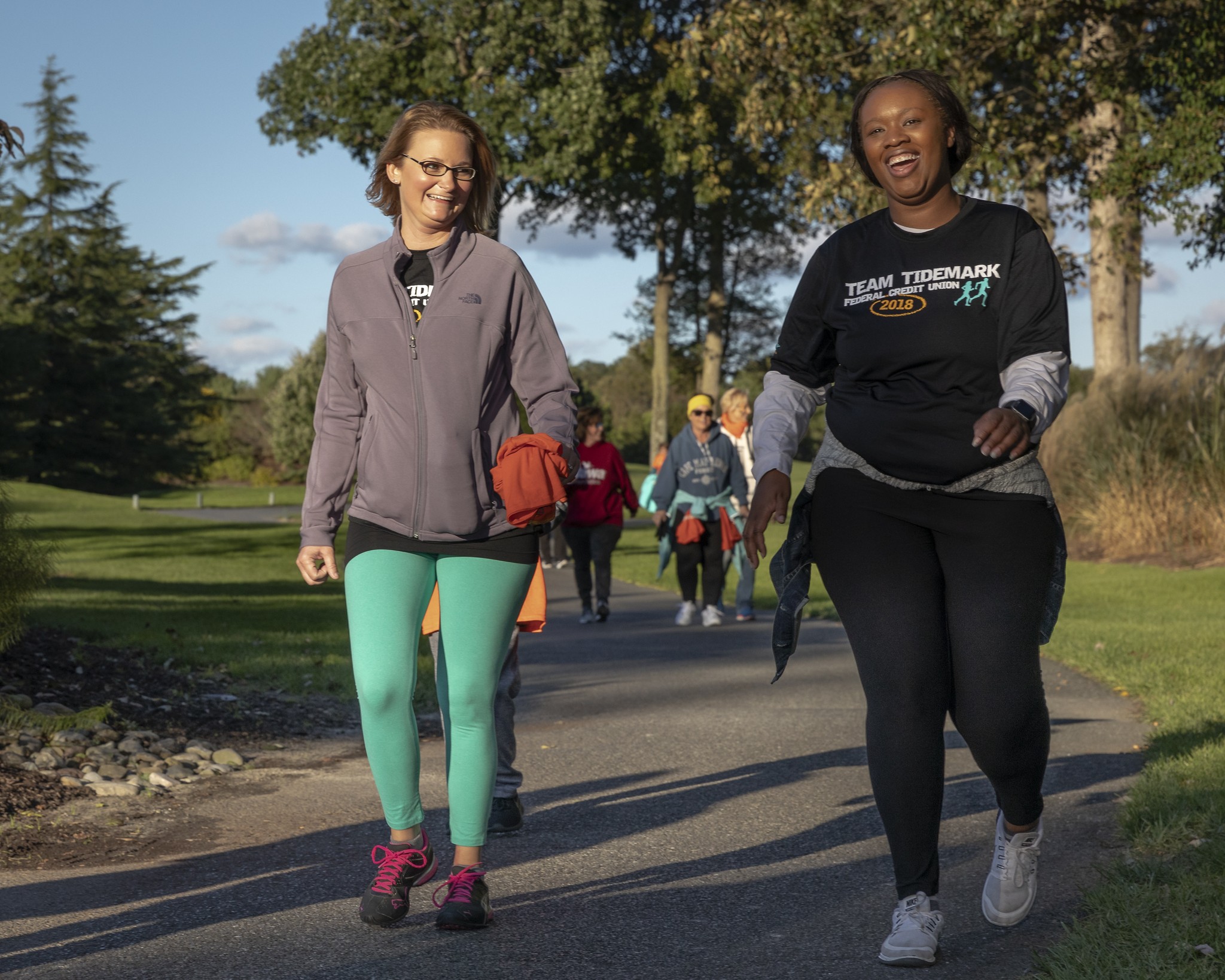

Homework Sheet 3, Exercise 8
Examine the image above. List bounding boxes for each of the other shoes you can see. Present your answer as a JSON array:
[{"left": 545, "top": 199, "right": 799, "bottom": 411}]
[
  {"left": 595, "top": 599, "right": 613, "bottom": 614},
  {"left": 580, "top": 611, "right": 595, "bottom": 625}
]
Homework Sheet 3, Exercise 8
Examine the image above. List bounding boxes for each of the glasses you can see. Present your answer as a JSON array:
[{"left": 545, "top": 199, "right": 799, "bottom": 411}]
[
  {"left": 591, "top": 421, "right": 605, "bottom": 427},
  {"left": 399, "top": 152, "right": 477, "bottom": 182},
  {"left": 692, "top": 409, "right": 713, "bottom": 417}
]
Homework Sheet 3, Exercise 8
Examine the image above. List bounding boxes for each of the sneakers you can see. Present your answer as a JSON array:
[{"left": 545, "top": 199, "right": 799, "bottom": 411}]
[
  {"left": 483, "top": 789, "right": 525, "bottom": 836},
  {"left": 355, "top": 822, "right": 438, "bottom": 928},
  {"left": 702, "top": 603, "right": 723, "bottom": 626},
  {"left": 882, "top": 891, "right": 947, "bottom": 961},
  {"left": 979, "top": 802, "right": 1044, "bottom": 925},
  {"left": 673, "top": 600, "right": 695, "bottom": 626},
  {"left": 430, "top": 862, "right": 493, "bottom": 933}
]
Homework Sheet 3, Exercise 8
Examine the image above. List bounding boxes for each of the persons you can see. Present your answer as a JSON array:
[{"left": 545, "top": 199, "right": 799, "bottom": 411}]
[
  {"left": 421, "top": 551, "right": 545, "bottom": 838},
  {"left": 653, "top": 515, "right": 672, "bottom": 580},
  {"left": 742, "top": 70, "right": 1074, "bottom": 965},
  {"left": 538, "top": 524, "right": 570, "bottom": 571},
  {"left": 714, "top": 387, "right": 756, "bottom": 622},
  {"left": 294, "top": 100, "right": 580, "bottom": 930},
  {"left": 650, "top": 441, "right": 668, "bottom": 475},
  {"left": 560, "top": 405, "right": 641, "bottom": 625},
  {"left": 650, "top": 393, "right": 750, "bottom": 627}
]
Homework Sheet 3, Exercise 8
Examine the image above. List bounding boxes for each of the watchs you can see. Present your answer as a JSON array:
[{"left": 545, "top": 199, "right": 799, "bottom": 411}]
[{"left": 1002, "top": 398, "right": 1037, "bottom": 431}]
